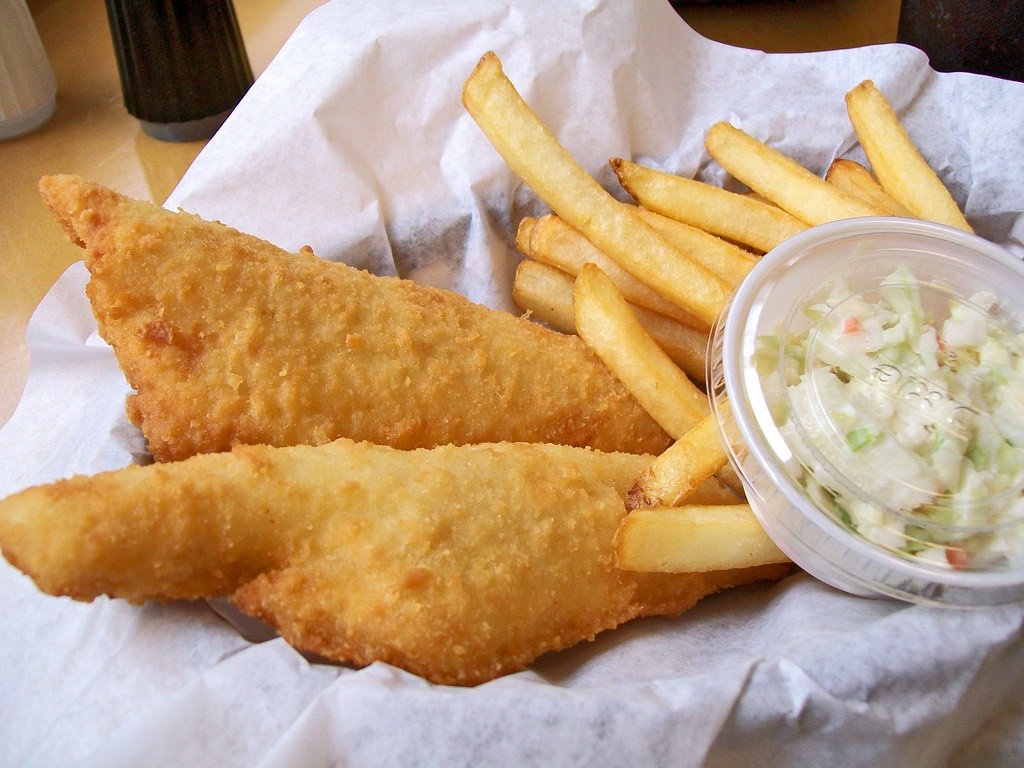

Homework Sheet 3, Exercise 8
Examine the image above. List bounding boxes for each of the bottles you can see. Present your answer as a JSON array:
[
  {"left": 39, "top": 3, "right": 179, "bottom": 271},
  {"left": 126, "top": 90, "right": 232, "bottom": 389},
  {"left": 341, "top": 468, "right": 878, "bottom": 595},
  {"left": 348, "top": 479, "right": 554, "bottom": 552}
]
[
  {"left": 0, "top": 0, "right": 58, "bottom": 142},
  {"left": 104, "top": 0, "right": 255, "bottom": 142}
]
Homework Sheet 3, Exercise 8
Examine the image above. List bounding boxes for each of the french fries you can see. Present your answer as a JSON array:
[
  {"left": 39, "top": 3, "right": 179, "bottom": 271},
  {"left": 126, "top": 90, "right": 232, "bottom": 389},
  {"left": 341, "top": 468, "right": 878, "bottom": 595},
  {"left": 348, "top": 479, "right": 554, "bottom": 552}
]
[{"left": 462, "top": 50, "right": 976, "bottom": 575}]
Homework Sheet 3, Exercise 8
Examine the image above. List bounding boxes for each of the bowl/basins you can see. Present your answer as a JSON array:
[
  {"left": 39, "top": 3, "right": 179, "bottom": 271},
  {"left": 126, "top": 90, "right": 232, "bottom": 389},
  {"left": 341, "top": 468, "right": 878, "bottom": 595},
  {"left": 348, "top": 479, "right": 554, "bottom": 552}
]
[{"left": 706, "top": 215, "right": 1023, "bottom": 608}]
